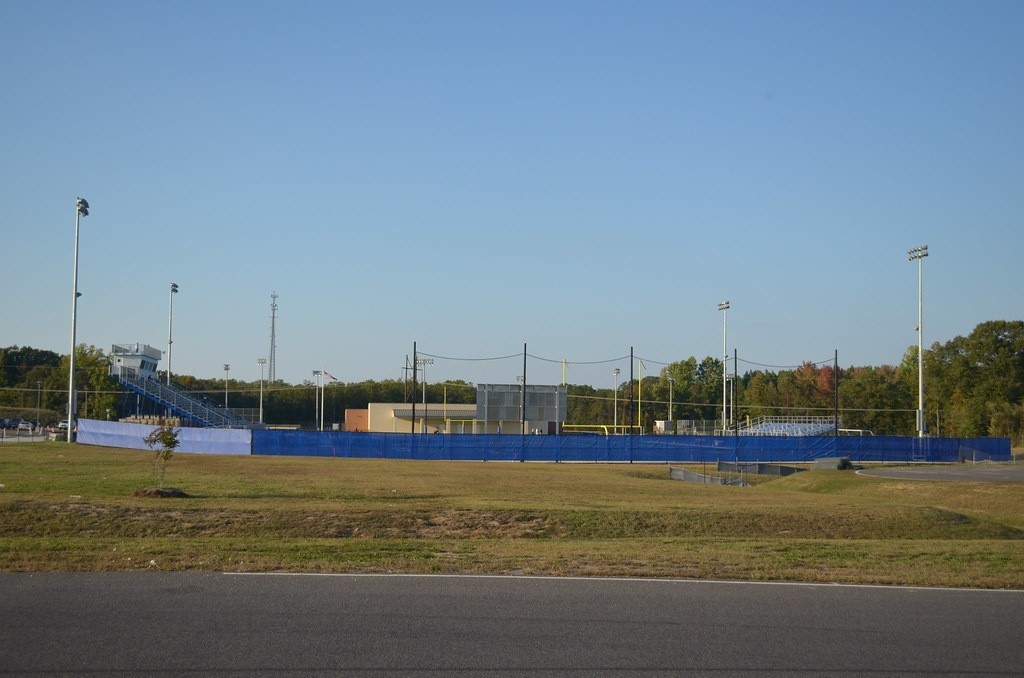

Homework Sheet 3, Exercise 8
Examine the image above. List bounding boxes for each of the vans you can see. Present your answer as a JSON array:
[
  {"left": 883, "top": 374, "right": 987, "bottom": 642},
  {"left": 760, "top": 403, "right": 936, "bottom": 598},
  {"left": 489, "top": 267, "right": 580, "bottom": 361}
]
[{"left": 4, "top": 418, "right": 25, "bottom": 429}]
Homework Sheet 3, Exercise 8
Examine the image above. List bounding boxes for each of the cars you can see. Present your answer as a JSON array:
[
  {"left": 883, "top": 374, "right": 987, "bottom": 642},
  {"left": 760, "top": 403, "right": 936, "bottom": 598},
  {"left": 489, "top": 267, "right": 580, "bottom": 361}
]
[
  {"left": 18, "top": 421, "right": 35, "bottom": 431},
  {"left": 59, "top": 420, "right": 76, "bottom": 430}
]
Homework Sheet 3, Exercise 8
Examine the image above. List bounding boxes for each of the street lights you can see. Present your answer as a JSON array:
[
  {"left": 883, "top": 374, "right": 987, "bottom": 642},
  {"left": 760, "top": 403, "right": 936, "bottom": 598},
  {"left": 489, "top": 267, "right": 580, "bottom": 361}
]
[
  {"left": 717, "top": 301, "right": 730, "bottom": 435},
  {"left": 416, "top": 358, "right": 435, "bottom": 433},
  {"left": 166, "top": 282, "right": 180, "bottom": 384},
  {"left": 905, "top": 243, "right": 929, "bottom": 436},
  {"left": 224, "top": 364, "right": 230, "bottom": 408},
  {"left": 256, "top": 357, "right": 267, "bottom": 422},
  {"left": 613, "top": 368, "right": 620, "bottom": 434},
  {"left": 66, "top": 198, "right": 90, "bottom": 442}
]
